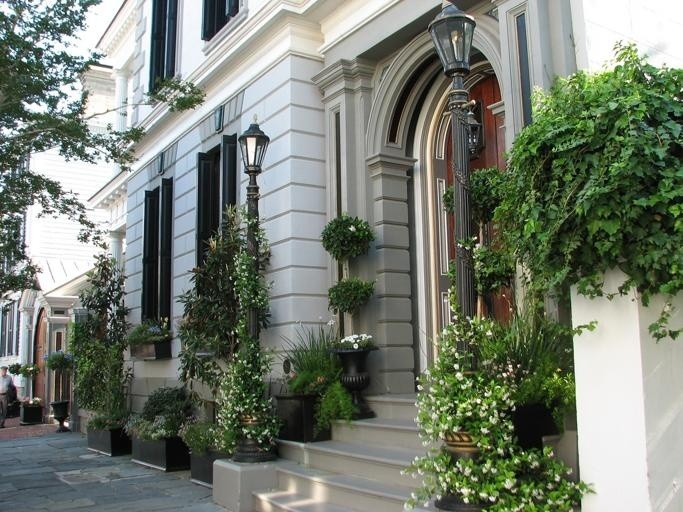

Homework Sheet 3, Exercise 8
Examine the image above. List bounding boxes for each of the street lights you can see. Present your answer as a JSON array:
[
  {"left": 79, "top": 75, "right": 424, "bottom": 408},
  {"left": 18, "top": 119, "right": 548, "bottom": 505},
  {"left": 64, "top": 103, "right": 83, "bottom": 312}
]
[
  {"left": 427, "top": 0, "right": 504, "bottom": 511},
  {"left": 231, "top": 113, "right": 277, "bottom": 464}
]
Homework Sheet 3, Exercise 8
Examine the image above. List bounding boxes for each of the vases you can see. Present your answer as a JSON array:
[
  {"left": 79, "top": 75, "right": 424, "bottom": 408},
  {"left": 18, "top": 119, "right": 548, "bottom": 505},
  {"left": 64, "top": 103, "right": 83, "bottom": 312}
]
[
  {"left": 49, "top": 400, "right": 72, "bottom": 433},
  {"left": 336, "top": 349, "right": 378, "bottom": 420},
  {"left": 18, "top": 405, "right": 42, "bottom": 425},
  {"left": 130, "top": 344, "right": 172, "bottom": 360},
  {"left": 275, "top": 391, "right": 331, "bottom": 443},
  {"left": 87, "top": 428, "right": 231, "bottom": 489}
]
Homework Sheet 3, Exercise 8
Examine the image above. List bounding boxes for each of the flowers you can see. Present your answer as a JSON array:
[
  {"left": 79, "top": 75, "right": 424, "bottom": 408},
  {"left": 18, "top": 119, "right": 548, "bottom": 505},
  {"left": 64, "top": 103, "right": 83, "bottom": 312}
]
[
  {"left": 340, "top": 333, "right": 375, "bottom": 350},
  {"left": 21, "top": 395, "right": 42, "bottom": 405},
  {"left": 128, "top": 320, "right": 171, "bottom": 343},
  {"left": 289, "top": 372, "right": 359, "bottom": 437},
  {"left": 86, "top": 408, "right": 232, "bottom": 457}
]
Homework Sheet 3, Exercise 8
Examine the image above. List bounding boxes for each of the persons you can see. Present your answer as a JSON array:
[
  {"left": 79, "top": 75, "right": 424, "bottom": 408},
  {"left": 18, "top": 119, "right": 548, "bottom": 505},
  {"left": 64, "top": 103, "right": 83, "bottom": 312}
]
[{"left": 0, "top": 366, "right": 12, "bottom": 428}]
